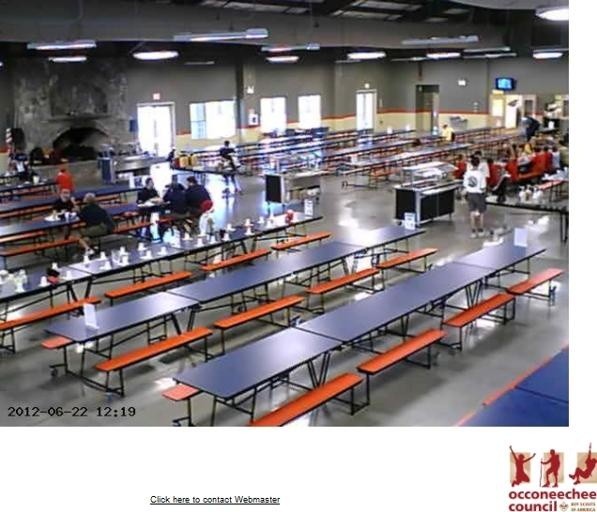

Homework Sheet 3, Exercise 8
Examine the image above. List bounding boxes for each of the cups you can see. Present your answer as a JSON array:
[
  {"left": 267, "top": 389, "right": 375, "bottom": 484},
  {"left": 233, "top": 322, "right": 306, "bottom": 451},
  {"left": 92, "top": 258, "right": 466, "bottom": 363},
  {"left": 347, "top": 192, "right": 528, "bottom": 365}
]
[
  {"left": 53, "top": 209, "right": 57, "bottom": 219},
  {"left": 0, "top": 216, "right": 265, "bottom": 293}
]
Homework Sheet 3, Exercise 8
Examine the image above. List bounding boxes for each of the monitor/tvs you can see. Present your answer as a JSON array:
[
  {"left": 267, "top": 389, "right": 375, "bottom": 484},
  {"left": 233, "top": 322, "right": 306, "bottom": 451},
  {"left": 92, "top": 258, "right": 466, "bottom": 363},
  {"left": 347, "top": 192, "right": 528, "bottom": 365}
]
[{"left": 496, "top": 77, "right": 514, "bottom": 90}]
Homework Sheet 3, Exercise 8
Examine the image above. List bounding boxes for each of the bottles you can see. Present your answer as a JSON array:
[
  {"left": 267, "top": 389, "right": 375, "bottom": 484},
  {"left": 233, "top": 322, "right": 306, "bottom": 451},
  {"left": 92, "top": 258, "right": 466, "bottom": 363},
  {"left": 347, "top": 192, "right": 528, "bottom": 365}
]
[{"left": 307, "top": 200, "right": 314, "bottom": 216}]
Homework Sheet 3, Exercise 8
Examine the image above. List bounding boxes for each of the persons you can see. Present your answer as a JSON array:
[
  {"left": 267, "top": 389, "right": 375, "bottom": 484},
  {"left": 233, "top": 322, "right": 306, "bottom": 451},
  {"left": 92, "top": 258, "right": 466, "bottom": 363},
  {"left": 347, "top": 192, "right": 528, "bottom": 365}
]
[
  {"left": 220, "top": 141, "right": 236, "bottom": 182},
  {"left": 453, "top": 129, "right": 569, "bottom": 198},
  {"left": 136, "top": 178, "right": 159, "bottom": 237},
  {"left": 52, "top": 189, "right": 75, "bottom": 239},
  {"left": 221, "top": 155, "right": 241, "bottom": 193},
  {"left": 163, "top": 175, "right": 192, "bottom": 232},
  {"left": 462, "top": 157, "right": 486, "bottom": 238},
  {"left": 55, "top": 167, "right": 74, "bottom": 194},
  {"left": 185, "top": 176, "right": 211, "bottom": 236},
  {"left": 166, "top": 148, "right": 175, "bottom": 168},
  {"left": 7, "top": 148, "right": 14, "bottom": 170},
  {"left": 443, "top": 124, "right": 454, "bottom": 143},
  {"left": 522, "top": 112, "right": 540, "bottom": 141},
  {"left": 75, "top": 192, "right": 113, "bottom": 257},
  {"left": 13, "top": 147, "right": 29, "bottom": 172}
]
